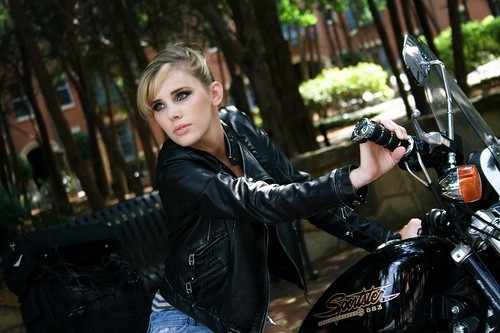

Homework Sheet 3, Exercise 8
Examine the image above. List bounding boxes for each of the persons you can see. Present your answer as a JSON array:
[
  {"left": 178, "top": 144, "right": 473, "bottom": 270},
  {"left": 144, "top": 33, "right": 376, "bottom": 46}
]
[{"left": 137, "top": 40, "right": 422, "bottom": 333}]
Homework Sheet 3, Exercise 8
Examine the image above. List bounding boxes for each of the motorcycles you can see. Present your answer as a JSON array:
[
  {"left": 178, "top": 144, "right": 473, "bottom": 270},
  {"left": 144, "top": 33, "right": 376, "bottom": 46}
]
[{"left": 3, "top": 32, "right": 500, "bottom": 333}]
